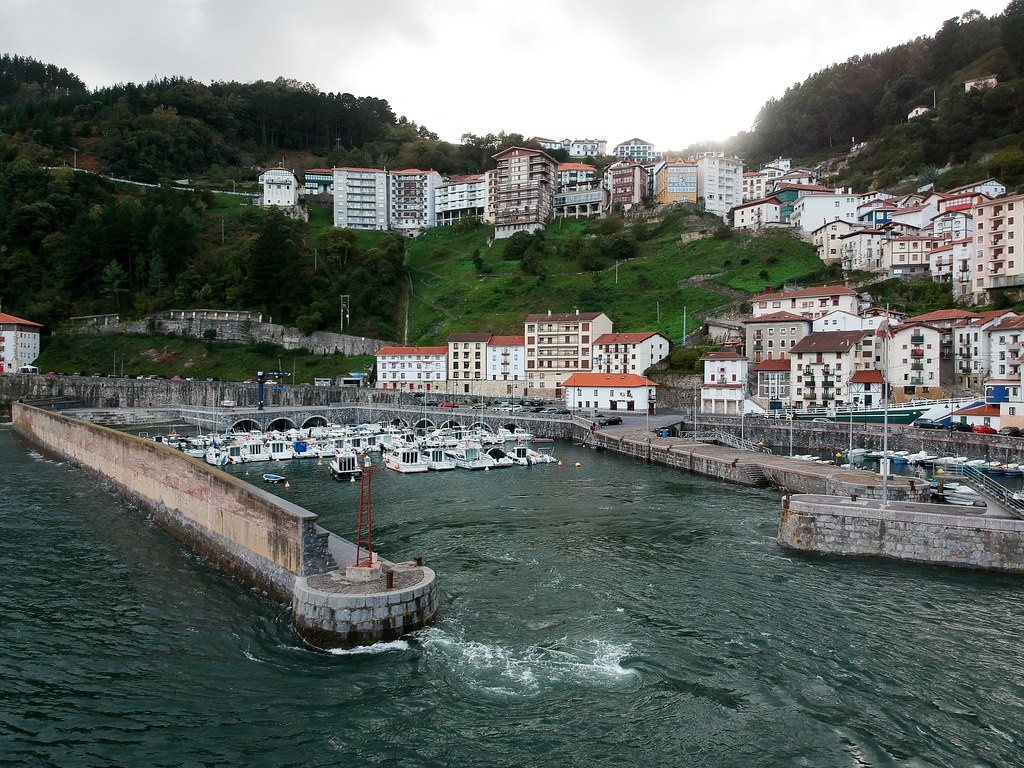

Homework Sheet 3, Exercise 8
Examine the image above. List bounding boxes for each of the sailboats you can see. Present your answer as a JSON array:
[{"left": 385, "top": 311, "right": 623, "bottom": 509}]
[
  {"left": 197, "top": 380, "right": 232, "bottom": 466},
  {"left": 414, "top": 384, "right": 494, "bottom": 471}
]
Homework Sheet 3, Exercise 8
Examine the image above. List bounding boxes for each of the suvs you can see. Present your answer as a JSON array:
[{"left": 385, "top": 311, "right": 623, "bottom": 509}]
[
  {"left": 524, "top": 400, "right": 532, "bottom": 405},
  {"left": 813, "top": 417, "right": 833, "bottom": 423},
  {"left": 914, "top": 418, "right": 943, "bottom": 429},
  {"left": 492, "top": 404, "right": 523, "bottom": 412},
  {"left": 534, "top": 399, "right": 543, "bottom": 406},
  {"left": 471, "top": 404, "right": 487, "bottom": 409}
]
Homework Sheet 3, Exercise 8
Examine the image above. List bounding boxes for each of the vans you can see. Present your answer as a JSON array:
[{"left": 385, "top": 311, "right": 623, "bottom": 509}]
[{"left": 502, "top": 400, "right": 509, "bottom": 407}]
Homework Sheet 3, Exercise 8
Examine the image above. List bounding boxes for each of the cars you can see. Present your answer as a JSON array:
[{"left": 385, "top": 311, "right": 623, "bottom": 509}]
[
  {"left": 528, "top": 407, "right": 545, "bottom": 412},
  {"left": 600, "top": 415, "right": 623, "bottom": 426},
  {"left": 420, "top": 400, "right": 438, "bottom": 407},
  {"left": 998, "top": 426, "right": 1022, "bottom": 437},
  {"left": 539, "top": 407, "right": 556, "bottom": 412},
  {"left": 972, "top": 425, "right": 996, "bottom": 434},
  {"left": 221, "top": 400, "right": 236, "bottom": 407},
  {"left": 46, "top": 370, "right": 279, "bottom": 385},
  {"left": 441, "top": 402, "right": 460, "bottom": 408},
  {"left": 911, "top": 396, "right": 930, "bottom": 402},
  {"left": 554, "top": 408, "right": 570, "bottom": 415},
  {"left": 948, "top": 422, "right": 972, "bottom": 432}
]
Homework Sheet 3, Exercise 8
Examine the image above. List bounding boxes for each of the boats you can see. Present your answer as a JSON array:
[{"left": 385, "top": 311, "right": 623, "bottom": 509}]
[
  {"left": 138, "top": 423, "right": 204, "bottom": 458},
  {"left": 263, "top": 473, "right": 285, "bottom": 484},
  {"left": 329, "top": 438, "right": 362, "bottom": 482},
  {"left": 483, "top": 442, "right": 558, "bottom": 468},
  {"left": 794, "top": 454, "right": 868, "bottom": 470},
  {"left": 222, "top": 410, "right": 415, "bottom": 462},
  {"left": 383, "top": 425, "right": 429, "bottom": 472},
  {"left": 846, "top": 444, "right": 1024, "bottom": 477},
  {"left": 395, "top": 407, "right": 535, "bottom": 448}
]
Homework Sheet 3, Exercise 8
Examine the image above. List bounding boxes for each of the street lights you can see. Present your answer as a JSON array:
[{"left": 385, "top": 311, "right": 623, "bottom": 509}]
[{"left": 643, "top": 376, "right": 648, "bottom": 430}]
